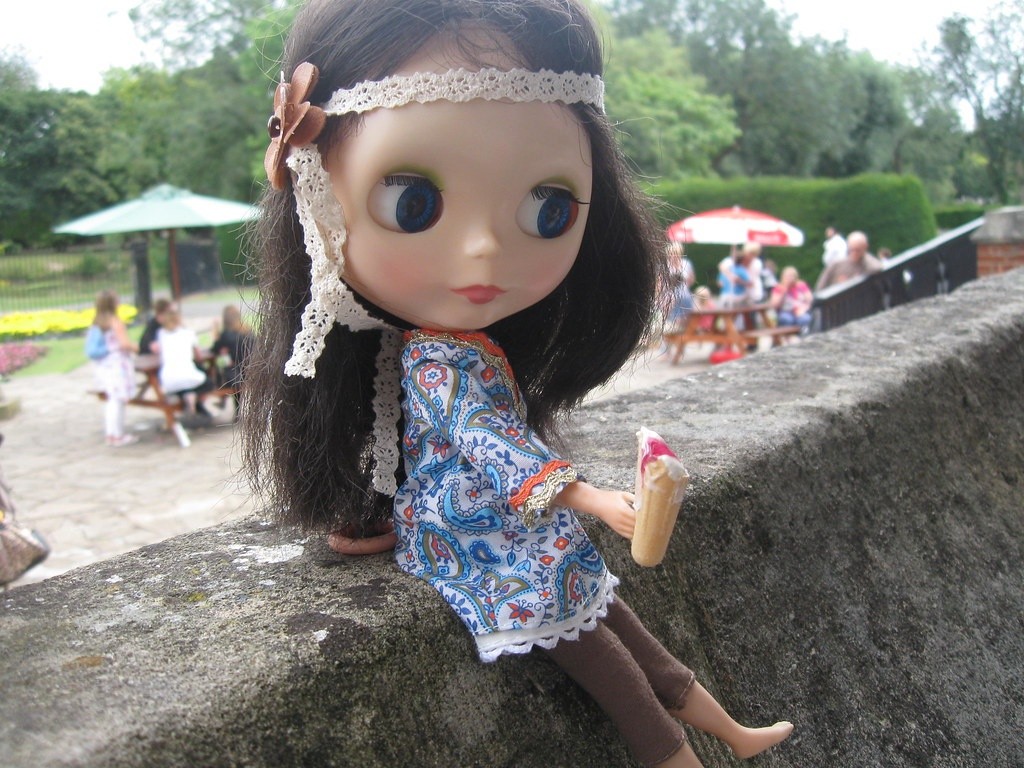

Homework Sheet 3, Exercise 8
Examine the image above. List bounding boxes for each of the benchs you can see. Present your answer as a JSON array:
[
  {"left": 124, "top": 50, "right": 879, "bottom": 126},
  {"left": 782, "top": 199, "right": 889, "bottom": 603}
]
[
  {"left": 93, "top": 380, "right": 238, "bottom": 438},
  {"left": 658, "top": 322, "right": 808, "bottom": 368}
]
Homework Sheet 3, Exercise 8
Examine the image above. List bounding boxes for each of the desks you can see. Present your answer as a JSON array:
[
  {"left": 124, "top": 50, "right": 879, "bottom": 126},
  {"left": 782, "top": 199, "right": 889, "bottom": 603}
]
[
  {"left": 91, "top": 345, "right": 244, "bottom": 438},
  {"left": 660, "top": 302, "right": 792, "bottom": 370}
]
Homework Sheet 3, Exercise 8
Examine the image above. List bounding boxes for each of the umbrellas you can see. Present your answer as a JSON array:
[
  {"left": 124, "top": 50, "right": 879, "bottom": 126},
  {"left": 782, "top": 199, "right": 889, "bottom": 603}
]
[
  {"left": 664, "top": 203, "right": 804, "bottom": 304},
  {"left": 54, "top": 183, "right": 266, "bottom": 306}
]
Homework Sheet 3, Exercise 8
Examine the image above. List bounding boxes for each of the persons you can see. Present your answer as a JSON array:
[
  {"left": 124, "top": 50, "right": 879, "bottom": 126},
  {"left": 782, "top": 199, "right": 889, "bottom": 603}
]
[
  {"left": 876, "top": 246, "right": 892, "bottom": 263},
  {"left": 231, "top": 0, "right": 796, "bottom": 768},
  {"left": 86, "top": 289, "right": 140, "bottom": 449},
  {"left": 655, "top": 241, "right": 812, "bottom": 359},
  {"left": 821, "top": 226, "right": 847, "bottom": 267},
  {"left": 815, "top": 231, "right": 880, "bottom": 292},
  {"left": 156, "top": 309, "right": 208, "bottom": 419},
  {"left": 210, "top": 304, "right": 258, "bottom": 409},
  {"left": 137, "top": 299, "right": 171, "bottom": 356}
]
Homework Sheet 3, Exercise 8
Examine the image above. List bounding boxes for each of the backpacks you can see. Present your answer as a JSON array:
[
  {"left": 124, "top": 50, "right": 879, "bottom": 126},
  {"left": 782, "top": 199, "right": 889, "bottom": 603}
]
[{"left": 86, "top": 326, "right": 108, "bottom": 358}]
